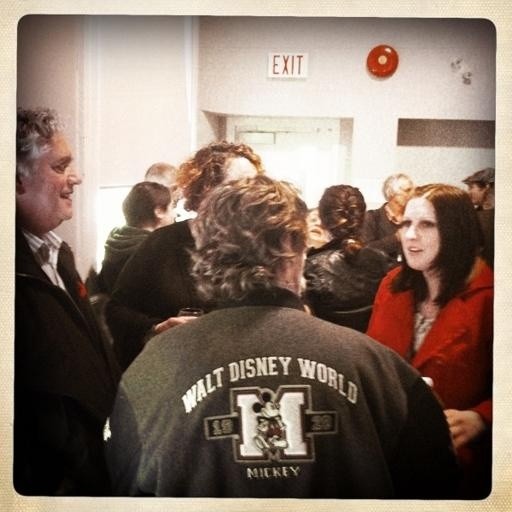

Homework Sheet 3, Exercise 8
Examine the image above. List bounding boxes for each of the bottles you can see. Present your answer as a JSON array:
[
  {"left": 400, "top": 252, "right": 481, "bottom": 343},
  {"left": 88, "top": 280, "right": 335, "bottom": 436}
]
[{"left": 423, "top": 377, "right": 449, "bottom": 415}]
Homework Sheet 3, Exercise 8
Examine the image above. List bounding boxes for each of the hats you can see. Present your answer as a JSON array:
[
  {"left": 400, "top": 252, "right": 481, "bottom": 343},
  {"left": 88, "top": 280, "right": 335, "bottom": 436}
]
[{"left": 461, "top": 162, "right": 494, "bottom": 184}]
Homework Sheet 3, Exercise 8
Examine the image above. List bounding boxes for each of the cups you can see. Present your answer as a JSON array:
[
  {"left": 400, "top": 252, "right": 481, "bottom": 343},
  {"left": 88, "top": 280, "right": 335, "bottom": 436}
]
[{"left": 178, "top": 307, "right": 203, "bottom": 321}]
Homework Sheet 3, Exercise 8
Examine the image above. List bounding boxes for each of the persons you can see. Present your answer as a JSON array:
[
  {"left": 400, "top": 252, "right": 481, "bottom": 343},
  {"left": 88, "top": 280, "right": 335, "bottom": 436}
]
[
  {"left": 102, "top": 174, "right": 464, "bottom": 497},
  {"left": 302, "top": 203, "right": 331, "bottom": 247},
  {"left": 304, "top": 182, "right": 399, "bottom": 334},
  {"left": 364, "top": 183, "right": 492, "bottom": 476},
  {"left": 362, "top": 172, "right": 416, "bottom": 259},
  {"left": 16, "top": 107, "right": 124, "bottom": 496},
  {"left": 146, "top": 161, "right": 184, "bottom": 211},
  {"left": 464, "top": 168, "right": 495, "bottom": 264},
  {"left": 105, "top": 138, "right": 314, "bottom": 374},
  {"left": 99, "top": 181, "right": 176, "bottom": 299}
]
